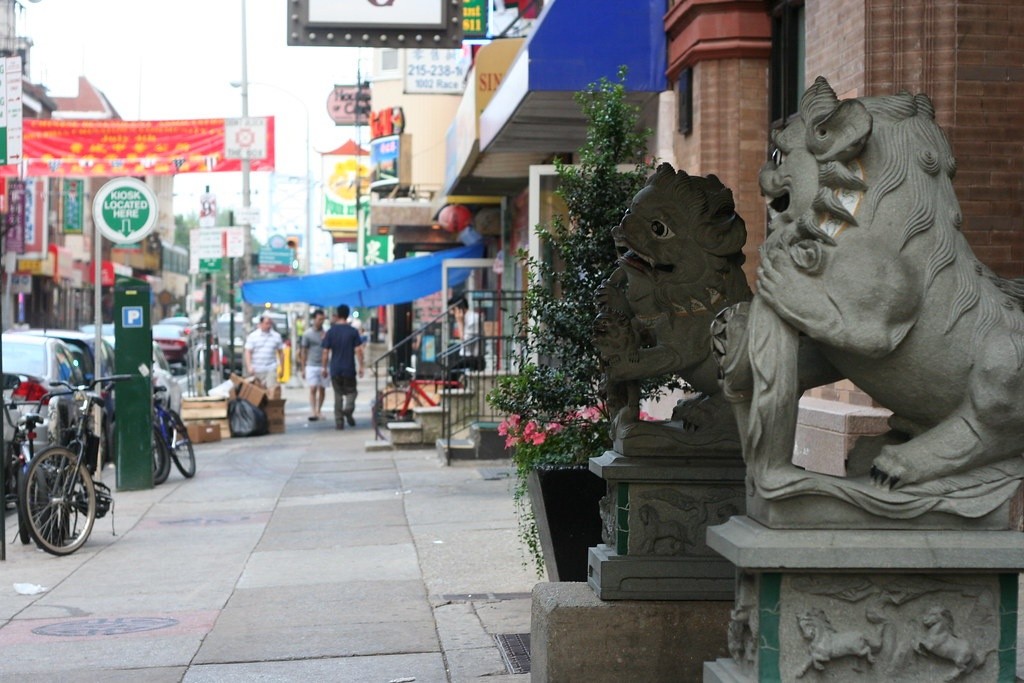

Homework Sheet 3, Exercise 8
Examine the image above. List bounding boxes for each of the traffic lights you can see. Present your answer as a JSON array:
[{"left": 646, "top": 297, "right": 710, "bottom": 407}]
[{"left": 287, "top": 236, "right": 297, "bottom": 249}]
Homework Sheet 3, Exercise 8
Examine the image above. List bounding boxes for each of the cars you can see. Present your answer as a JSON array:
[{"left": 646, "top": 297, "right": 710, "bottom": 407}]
[
  {"left": 2, "top": 335, "right": 86, "bottom": 462},
  {"left": 3, "top": 312, "right": 289, "bottom": 476}
]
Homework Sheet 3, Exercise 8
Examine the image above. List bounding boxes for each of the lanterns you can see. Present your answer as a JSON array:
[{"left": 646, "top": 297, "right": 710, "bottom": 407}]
[{"left": 439, "top": 205, "right": 471, "bottom": 232}]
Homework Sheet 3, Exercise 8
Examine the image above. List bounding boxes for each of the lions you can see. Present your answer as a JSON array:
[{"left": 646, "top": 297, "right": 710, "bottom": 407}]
[
  {"left": 757, "top": 75, "right": 1024, "bottom": 486},
  {"left": 590, "top": 159, "right": 758, "bottom": 430}
]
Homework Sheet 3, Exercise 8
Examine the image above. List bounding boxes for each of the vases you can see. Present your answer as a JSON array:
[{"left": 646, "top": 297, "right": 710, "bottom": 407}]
[{"left": 526, "top": 465, "right": 606, "bottom": 582}]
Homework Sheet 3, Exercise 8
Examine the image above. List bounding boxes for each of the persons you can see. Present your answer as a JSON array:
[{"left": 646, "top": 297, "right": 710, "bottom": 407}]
[
  {"left": 321, "top": 305, "right": 364, "bottom": 430},
  {"left": 445, "top": 296, "right": 486, "bottom": 390},
  {"left": 296, "top": 310, "right": 332, "bottom": 421},
  {"left": 359, "top": 326, "right": 367, "bottom": 342},
  {"left": 330, "top": 314, "right": 336, "bottom": 326},
  {"left": 245, "top": 315, "right": 285, "bottom": 388}
]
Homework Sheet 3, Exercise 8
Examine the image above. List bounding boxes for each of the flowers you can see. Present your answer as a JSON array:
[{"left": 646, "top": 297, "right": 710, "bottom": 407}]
[{"left": 484, "top": 65, "right": 664, "bottom": 583}]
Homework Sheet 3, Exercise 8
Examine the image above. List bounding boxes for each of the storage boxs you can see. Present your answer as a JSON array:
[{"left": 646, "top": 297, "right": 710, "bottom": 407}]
[{"left": 180, "top": 370, "right": 286, "bottom": 444}]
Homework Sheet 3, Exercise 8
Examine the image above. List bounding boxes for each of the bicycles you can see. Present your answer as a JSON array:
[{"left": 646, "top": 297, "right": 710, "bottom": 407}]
[
  {"left": 19, "top": 374, "right": 133, "bottom": 556},
  {"left": 371, "top": 362, "right": 470, "bottom": 442},
  {"left": 108, "top": 385, "right": 196, "bottom": 485},
  {"left": 2, "top": 388, "right": 75, "bottom": 545}
]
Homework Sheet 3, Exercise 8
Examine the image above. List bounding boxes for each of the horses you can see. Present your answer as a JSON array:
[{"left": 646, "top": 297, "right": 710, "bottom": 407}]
[
  {"left": 795, "top": 606, "right": 885, "bottom": 679},
  {"left": 636, "top": 505, "right": 689, "bottom": 555},
  {"left": 914, "top": 607, "right": 989, "bottom": 683}
]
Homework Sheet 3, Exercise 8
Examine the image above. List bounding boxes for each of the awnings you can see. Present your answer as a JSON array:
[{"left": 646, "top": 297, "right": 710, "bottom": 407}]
[{"left": 479, "top": 0, "right": 668, "bottom": 153}]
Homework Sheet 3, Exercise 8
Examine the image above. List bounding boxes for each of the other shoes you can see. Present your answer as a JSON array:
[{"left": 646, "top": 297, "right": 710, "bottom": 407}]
[
  {"left": 345, "top": 412, "right": 354, "bottom": 426},
  {"left": 309, "top": 414, "right": 324, "bottom": 420},
  {"left": 336, "top": 424, "right": 343, "bottom": 429}
]
